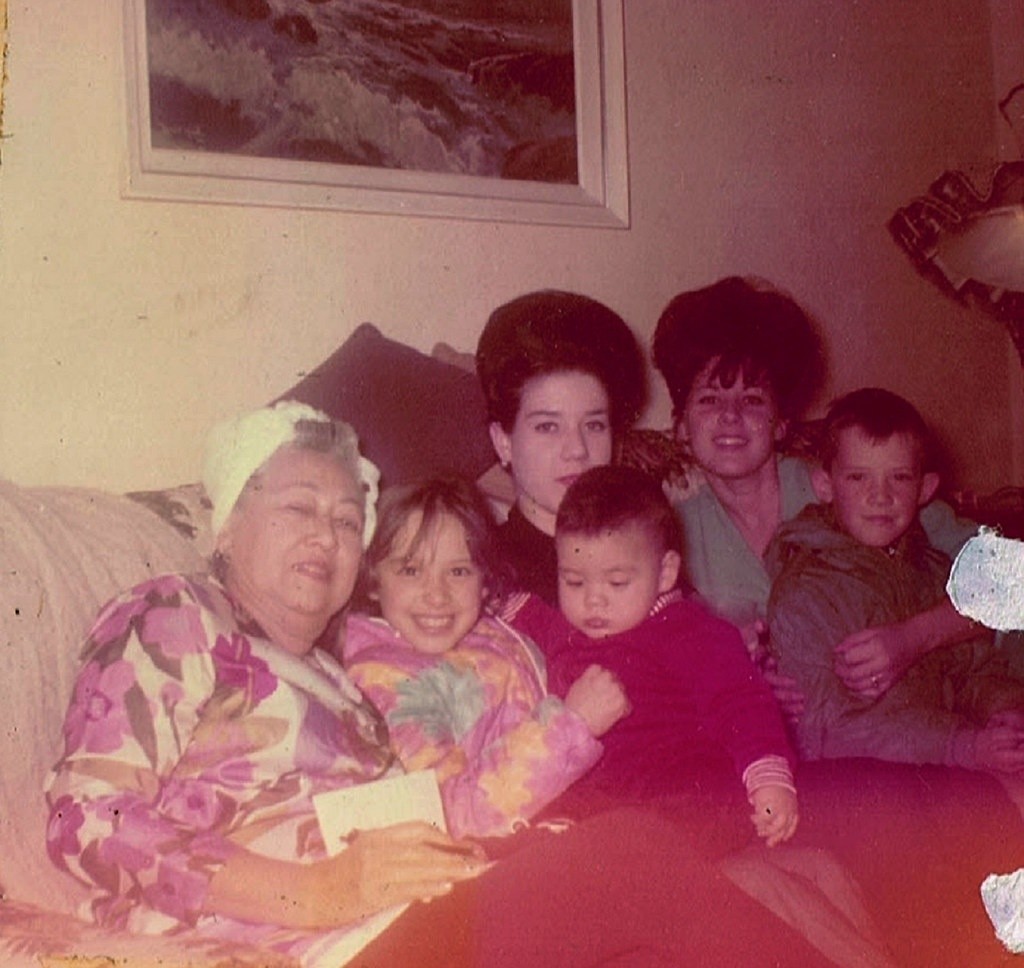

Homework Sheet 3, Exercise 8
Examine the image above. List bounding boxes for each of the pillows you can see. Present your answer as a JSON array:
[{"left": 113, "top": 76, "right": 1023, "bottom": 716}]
[{"left": 258, "top": 314, "right": 523, "bottom": 520}]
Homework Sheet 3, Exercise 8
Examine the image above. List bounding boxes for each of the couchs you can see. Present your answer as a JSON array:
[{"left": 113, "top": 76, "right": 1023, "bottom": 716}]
[{"left": 0, "top": 419, "right": 843, "bottom": 968}]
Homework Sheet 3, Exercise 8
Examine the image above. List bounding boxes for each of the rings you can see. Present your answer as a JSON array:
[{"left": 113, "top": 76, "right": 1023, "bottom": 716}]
[{"left": 869, "top": 676, "right": 879, "bottom": 688}]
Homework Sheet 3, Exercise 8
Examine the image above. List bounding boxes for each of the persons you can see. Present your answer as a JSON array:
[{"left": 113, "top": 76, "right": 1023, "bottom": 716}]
[
  {"left": 475, "top": 275, "right": 1024, "bottom": 968},
  {"left": 331, "top": 466, "right": 629, "bottom": 850},
  {"left": 47, "top": 400, "right": 833, "bottom": 968},
  {"left": 489, "top": 464, "right": 795, "bottom": 858}
]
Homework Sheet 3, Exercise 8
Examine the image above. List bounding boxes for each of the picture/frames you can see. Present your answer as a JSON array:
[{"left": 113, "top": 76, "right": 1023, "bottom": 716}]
[{"left": 117, "top": 0, "right": 632, "bottom": 231}]
